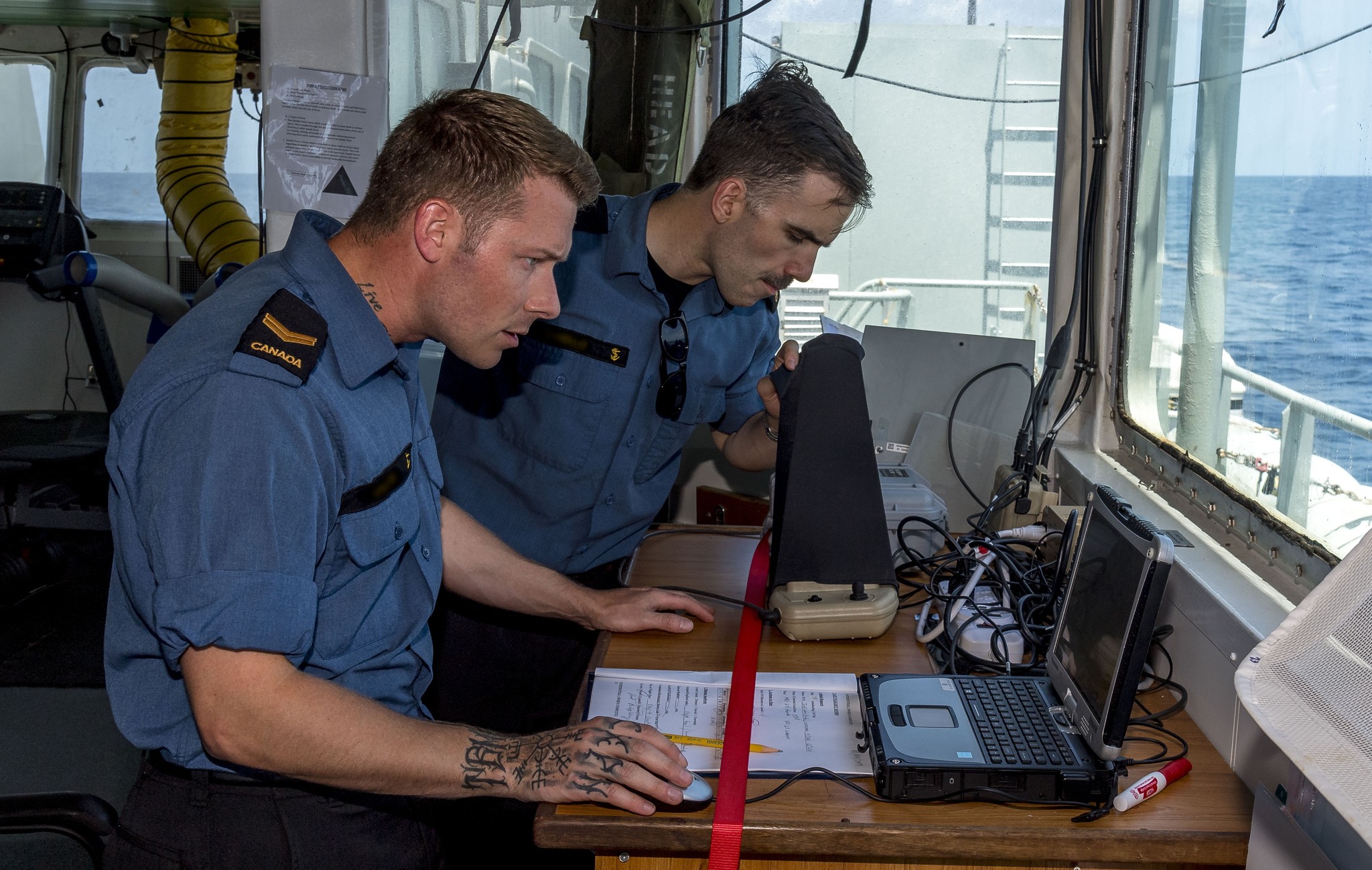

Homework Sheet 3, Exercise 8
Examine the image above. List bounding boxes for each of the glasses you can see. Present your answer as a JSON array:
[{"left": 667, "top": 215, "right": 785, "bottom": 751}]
[{"left": 654, "top": 309, "right": 691, "bottom": 424}]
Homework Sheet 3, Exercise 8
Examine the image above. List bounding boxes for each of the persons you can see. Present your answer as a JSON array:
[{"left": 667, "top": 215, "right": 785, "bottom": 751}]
[
  {"left": 424, "top": 63, "right": 871, "bottom": 870},
  {"left": 101, "top": 87, "right": 715, "bottom": 870}
]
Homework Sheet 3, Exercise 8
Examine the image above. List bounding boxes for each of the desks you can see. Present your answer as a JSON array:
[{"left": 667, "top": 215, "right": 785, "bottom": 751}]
[{"left": 534, "top": 522, "right": 1255, "bottom": 870}]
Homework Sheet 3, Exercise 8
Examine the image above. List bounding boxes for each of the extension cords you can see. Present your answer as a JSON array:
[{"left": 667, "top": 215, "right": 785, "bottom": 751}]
[{"left": 933, "top": 580, "right": 1024, "bottom": 665}]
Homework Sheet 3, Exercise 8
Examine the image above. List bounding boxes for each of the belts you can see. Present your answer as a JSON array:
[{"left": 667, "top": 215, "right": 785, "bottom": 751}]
[{"left": 140, "top": 747, "right": 289, "bottom": 787}]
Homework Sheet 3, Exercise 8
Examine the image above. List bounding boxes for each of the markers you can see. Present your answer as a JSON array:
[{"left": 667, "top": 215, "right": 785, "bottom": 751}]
[{"left": 1113, "top": 758, "right": 1195, "bottom": 812}]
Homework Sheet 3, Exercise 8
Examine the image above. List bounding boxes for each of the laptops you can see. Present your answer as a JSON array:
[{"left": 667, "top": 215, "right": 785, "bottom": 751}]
[{"left": 858, "top": 483, "right": 1173, "bottom": 802}]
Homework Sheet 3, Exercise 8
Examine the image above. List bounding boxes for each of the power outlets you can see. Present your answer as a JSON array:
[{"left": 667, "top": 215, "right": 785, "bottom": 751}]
[{"left": 85, "top": 364, "right": 101, "bottom": 388}]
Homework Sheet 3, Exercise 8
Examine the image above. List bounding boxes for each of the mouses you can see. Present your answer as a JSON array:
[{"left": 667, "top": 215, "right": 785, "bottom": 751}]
[{"left": 595, "top": 762, "right": 714, "bottom": 813}]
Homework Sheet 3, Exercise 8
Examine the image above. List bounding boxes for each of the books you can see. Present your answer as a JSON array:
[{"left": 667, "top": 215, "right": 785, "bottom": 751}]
[{"left": 586, "top": 668, "right": 872, "bottom": 773}]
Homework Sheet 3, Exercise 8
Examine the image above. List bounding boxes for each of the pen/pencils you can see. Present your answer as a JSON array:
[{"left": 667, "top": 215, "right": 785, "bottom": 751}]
[{"left": 660, "top": 732, "right": 783, "bottom": 753}]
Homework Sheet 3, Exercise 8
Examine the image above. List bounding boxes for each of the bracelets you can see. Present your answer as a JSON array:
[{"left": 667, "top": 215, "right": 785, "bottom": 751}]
[{"left": 762, "top": 412, "right": 778, "bottom": 442}]
